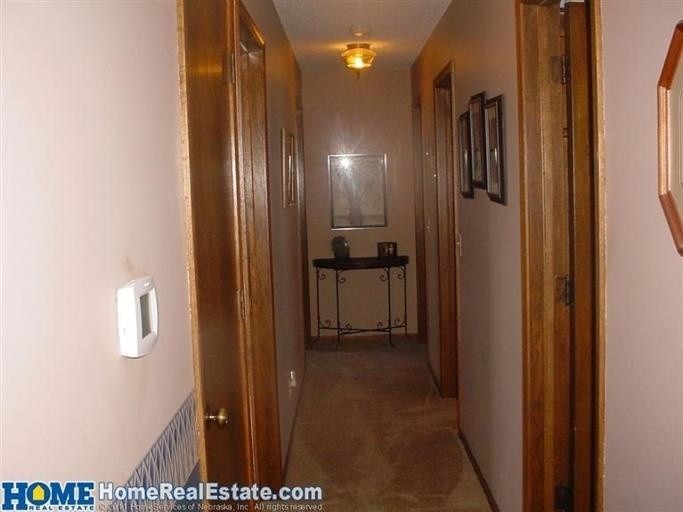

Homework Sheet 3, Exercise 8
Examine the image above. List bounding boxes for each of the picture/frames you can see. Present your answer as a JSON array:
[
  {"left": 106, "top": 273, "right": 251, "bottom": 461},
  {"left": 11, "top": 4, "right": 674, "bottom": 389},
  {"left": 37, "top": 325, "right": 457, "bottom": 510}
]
[
  {"left": 456, "top": 110, "right": 475, "bottom": 199},
  {"left": 280, "top": 130, "right": 301, "bottom": 211},
  {"left": 654, "top": 19, "right": 683, "bottom": 258},
  {"left": 324, "top": 151, "right": 393, "bottom": 234},
  {"left": 482, "top": 93, "right": 509, "bottom": 205},
  {"left": 467, "top": 90, "right": 486, "bottom": 190}
]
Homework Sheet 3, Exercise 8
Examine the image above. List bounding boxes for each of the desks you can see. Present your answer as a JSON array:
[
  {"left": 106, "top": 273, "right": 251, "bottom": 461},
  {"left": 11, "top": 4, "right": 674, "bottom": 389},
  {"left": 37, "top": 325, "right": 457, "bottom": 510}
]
[{"left": 311, "top": 252, "right": 410, "bottom": 352}]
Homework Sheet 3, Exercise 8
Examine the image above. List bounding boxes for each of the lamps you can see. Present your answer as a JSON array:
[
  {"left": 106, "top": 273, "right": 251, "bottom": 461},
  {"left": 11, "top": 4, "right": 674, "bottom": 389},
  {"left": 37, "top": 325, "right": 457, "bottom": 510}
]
[{"left": 335, "top": 29, "right": 376, "bottom": 76}]
